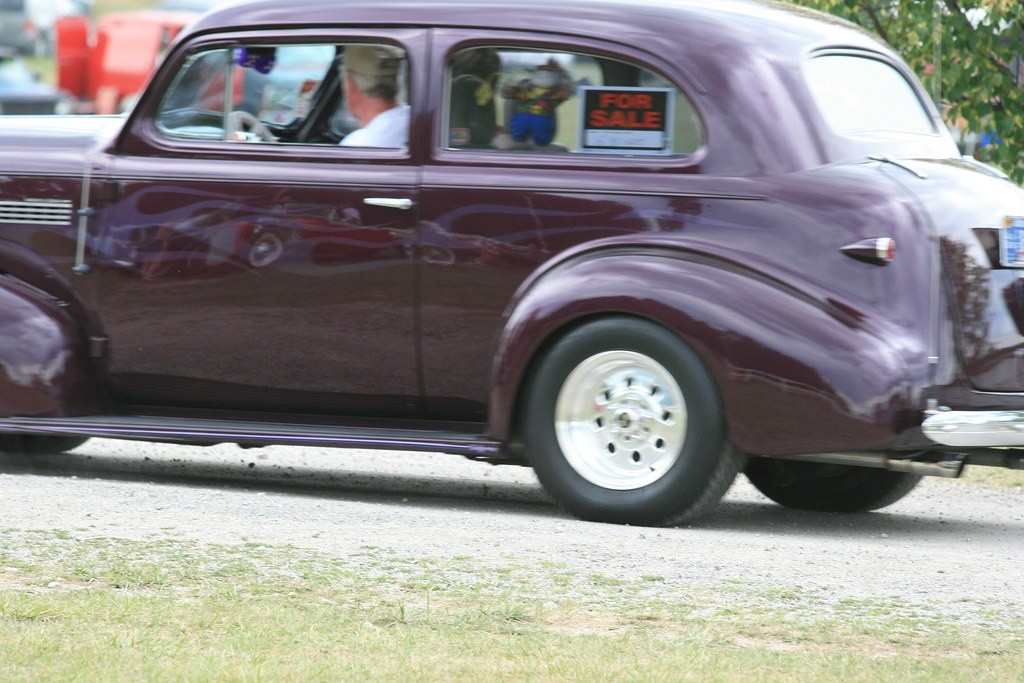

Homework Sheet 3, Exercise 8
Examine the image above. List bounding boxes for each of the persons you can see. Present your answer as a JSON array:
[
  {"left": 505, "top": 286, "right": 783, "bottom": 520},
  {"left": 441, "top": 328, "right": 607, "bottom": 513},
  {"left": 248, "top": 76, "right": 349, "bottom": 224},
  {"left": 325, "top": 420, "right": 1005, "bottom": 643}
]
[{"left": 228, "top": 43, "right": 410, "bottom": 151}]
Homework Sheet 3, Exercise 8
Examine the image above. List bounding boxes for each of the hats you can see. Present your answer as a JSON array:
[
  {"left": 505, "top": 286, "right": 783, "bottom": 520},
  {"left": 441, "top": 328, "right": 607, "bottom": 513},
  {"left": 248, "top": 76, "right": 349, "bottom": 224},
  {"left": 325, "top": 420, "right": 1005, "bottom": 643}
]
[{"left": 336, "top": 44, "right": 406, "bottom": 78}]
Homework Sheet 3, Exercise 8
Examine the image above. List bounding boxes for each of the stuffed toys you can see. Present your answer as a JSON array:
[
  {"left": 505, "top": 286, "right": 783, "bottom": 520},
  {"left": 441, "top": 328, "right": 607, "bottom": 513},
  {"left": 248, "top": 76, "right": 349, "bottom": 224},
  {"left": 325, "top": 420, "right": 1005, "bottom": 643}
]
[{"left": 493, "top": 63, "right": 588, "bottom": 151}]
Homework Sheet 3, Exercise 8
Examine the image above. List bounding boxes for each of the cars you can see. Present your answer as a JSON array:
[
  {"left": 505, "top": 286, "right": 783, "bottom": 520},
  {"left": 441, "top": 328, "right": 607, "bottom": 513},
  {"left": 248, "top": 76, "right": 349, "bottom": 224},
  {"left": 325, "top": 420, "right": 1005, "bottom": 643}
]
[{"left": 0, "top": 0, "right": 1024, "bottom": 528}]
